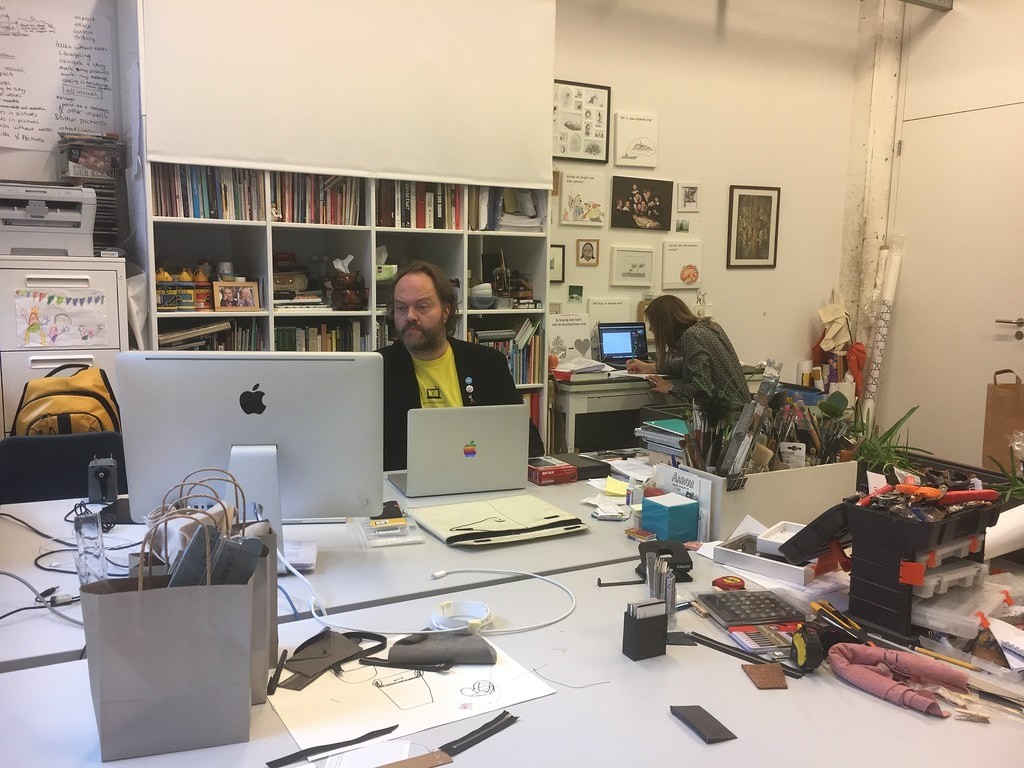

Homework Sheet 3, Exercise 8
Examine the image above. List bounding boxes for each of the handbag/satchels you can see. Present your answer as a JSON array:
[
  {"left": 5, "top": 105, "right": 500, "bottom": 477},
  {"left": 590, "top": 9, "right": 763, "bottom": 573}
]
[
  {"left": 77, "top": 469, "right": 277, "bottom": 762},
  {"left": 982, "top": 369, "right": 1024, "bottom": 477}
]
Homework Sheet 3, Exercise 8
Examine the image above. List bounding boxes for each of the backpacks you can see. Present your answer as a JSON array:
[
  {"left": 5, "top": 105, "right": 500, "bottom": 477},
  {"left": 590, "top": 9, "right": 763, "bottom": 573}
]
[{"left": 10, "top": 363, "right": 120, "bottom": 435}]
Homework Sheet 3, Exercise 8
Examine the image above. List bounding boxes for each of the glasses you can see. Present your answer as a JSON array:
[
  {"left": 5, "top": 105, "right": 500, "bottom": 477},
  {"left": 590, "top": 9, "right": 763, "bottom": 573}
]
[{"left": 649, "top": 323, "right": 654, "bottom": 332}]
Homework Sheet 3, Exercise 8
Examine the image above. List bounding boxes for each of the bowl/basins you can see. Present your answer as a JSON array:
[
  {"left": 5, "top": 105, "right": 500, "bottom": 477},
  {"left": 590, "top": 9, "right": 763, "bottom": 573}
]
[{"left": 468, "top": 282, "right": 495, "bottom": 309}]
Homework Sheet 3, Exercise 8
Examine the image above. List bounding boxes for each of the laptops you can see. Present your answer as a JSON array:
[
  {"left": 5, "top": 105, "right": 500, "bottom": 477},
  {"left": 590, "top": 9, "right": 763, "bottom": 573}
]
[
  {"left": 387, "top": 405, "right": 528, "bottom": 496},
  {"left": 595, "top": 323, "right": 654, "bottom": 370}
]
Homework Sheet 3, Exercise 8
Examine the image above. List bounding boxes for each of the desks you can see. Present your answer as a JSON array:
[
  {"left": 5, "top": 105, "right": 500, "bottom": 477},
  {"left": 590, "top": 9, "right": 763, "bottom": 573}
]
[{"left": 0, "top": 366, "right": 1022, "bottom": 768}]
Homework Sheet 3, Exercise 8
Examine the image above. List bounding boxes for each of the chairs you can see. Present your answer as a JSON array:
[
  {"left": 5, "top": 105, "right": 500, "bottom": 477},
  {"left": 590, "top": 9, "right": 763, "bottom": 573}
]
[{"left": 0, "top": 434, "right": 128, "bottom": 503}]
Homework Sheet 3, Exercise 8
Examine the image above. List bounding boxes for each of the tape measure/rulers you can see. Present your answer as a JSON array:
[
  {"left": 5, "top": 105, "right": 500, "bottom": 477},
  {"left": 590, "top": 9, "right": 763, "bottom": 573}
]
[
  {"left": 719, "top": 403, "right": 756, "bottom": 476},
  {"left": 789, "top": 623, "right": 824, "bottom": 672},
  {"left": 712, "top": 576, "right": 747, "bottom": 590}
]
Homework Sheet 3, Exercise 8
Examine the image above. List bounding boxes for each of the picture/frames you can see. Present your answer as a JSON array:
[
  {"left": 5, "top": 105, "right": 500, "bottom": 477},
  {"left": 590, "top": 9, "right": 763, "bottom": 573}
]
[
  {"left": 559, "top": 172, "right": 608, "bottom": 227},
  {"left": 726, "top": 185, "right": 781, "bottom": 268},
  {"left": 576, "top": 238, "right": 600, "bottom": 266},
  {"left": 677, "top": 183, "right": 701, "bottom": 212},
  {"left": 552, "top": 79, "right": 611, "bottom": 163},
  {"left": 610, "top": 247, "right": 655, "bottom": 287},
  {"left": 550, "top": 245, "right": 565, "bottom": 283}
]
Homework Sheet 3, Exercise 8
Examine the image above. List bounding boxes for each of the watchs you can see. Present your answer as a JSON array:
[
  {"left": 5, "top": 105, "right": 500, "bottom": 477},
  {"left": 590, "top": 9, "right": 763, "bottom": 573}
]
[{"left": 669, "top": 385, "right": 675, "bottom": 391}]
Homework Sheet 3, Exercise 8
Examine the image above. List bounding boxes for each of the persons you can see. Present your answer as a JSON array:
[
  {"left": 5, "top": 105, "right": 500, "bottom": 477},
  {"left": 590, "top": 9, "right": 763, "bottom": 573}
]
[
  {"left": 372, "top": 262, "right": 545, "bottom": 472},
  {"left": 219, "top": 287, "right": 237, "bottom": 306},
  {"left": 616, "top": 185, "right": 660, "bottom": 217},
  {"left": 626, "top": 295, "right": 752, "bottom": 405},
  {"left": 238, "top": 287, "right": 255, "bottom": 307}
]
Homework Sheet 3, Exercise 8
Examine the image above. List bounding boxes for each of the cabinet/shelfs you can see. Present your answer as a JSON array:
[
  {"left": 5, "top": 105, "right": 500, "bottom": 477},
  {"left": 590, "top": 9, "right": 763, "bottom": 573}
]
[
  {"left": 122, "top": 163, "right": 552, "bottom": 453},
  {"left": 848, "top": 531, "right": 1012, "bottom": 645}
]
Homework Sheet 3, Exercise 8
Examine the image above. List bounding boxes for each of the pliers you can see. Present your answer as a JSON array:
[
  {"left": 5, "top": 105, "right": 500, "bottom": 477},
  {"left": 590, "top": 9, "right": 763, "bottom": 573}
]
[{"left": 810, "top": 599, "right": 878, "bottom": 647}]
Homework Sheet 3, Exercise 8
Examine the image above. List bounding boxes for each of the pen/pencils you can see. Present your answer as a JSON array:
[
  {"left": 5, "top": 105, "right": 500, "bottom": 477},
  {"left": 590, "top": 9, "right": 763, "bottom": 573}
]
[
  {"left": 266, "top": 649, "right": 288, "bottom": 695},
  {"left": 631, "top": 343, "right": 639, "bottom": 364},
  {"left": 908, "top": 645, "right": 990, "bottom": 674}
]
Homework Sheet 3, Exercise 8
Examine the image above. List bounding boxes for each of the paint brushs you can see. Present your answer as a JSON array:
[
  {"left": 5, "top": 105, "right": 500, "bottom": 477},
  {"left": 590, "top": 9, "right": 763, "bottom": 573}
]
[
  {"left": 685, "top": 434, "right": 694, "bottom": 466},
  {"left": 822, "top": 416, "right": 847, "bottom": 463},
  {"left": 694, "top": 409, "right": 721, "bottom": 466},
  {"left": 797, "top": 400, "right": 822, "bottom": 456},
  {"left": 766, "top": 406, "right": 797, "bottom": 460},
  {"left": 679, "top": 439, "right": 688, "bottom": 466}
]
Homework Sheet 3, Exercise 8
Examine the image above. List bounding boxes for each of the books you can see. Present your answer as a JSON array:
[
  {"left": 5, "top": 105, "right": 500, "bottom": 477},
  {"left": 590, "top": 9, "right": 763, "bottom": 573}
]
[
  {"left": 150, "top": 162, "right": 544, "bottom": 385},
  {"left": 633, "top": 418, "right": 689, "bottom": 467}
]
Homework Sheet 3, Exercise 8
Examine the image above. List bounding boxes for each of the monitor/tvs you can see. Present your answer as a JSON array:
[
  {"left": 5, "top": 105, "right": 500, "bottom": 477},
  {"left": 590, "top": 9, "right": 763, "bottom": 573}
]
[{"left": 114, "top": 349, "right": 383, "bottom": 573}]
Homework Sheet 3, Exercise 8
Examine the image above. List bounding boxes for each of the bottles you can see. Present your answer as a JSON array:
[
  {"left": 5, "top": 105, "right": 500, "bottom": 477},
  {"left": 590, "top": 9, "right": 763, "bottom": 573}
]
[{"left": 156, "top": 268, "right": 214, "bottom": 312}]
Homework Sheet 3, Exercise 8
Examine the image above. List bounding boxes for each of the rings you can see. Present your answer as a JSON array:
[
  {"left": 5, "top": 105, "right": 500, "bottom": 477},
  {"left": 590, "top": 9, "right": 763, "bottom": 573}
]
[{"left": 654, "top": 382, "right": 656, "bottom": 384}]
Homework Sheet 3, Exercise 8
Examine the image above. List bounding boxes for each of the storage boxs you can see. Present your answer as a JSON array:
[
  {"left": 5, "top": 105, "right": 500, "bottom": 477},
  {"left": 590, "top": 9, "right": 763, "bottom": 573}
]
[
  {"left": 843, "top": 494, "right": 1004, "bottom": 551},
  {"left": 679, "top": 460, "right": 857, "bottom": 540}
]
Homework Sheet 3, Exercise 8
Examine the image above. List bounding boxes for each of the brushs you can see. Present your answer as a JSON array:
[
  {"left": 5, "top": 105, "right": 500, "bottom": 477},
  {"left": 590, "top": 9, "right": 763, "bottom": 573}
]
[{"left": 749, "top": 442, "right": 774, "bottom": 471}]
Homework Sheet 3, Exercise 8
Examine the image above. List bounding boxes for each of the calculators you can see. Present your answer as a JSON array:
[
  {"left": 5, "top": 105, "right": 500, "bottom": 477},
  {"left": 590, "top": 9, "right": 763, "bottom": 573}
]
[{"left": 697, "top": 592, "right": 807, "bottom": 629}]
